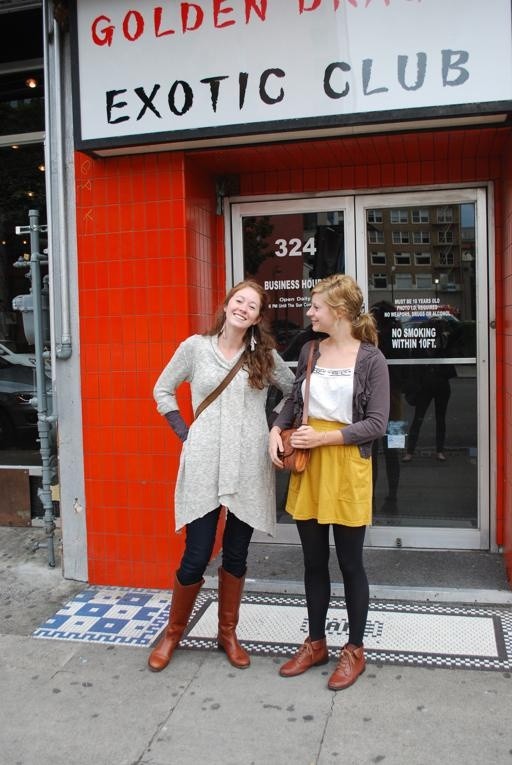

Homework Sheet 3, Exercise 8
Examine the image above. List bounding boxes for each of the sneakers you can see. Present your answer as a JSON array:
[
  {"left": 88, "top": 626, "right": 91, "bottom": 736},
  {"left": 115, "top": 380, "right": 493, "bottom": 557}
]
[
  {"left": 437, "top": 452, "right": 446, "bottom": 460},
  {"left": 379, "top": 498, "right": 398, "bottom": 514},
  {"left": 402, "top": 454, "right": 413, "bottom": 462}
]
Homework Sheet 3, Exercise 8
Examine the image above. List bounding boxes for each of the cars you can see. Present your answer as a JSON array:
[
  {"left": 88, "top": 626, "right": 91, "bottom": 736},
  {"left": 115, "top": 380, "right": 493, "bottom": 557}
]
[{"left": 0, "top": 342, "right": 52, "bottom": 450}]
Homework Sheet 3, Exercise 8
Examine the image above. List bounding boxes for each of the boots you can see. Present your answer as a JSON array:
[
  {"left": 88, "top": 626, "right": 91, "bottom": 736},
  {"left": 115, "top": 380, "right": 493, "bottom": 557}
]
[
  {"left": 147, "top": 570, "right": 205, "bottom": 673},
  {"left": 218, "top": 565, "right": 250, "bottom": 669}
]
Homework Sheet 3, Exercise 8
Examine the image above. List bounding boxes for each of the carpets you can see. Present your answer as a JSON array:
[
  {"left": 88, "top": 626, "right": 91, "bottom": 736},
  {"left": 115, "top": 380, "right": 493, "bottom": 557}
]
[{"left": 32, "top": 586, "right": 511, "bottom": 677}]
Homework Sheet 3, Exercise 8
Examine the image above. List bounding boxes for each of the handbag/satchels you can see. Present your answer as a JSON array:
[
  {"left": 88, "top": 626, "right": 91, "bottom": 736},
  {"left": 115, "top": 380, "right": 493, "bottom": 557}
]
[{"left": 277, "top": 429, "right": 312, "bottom": 474}]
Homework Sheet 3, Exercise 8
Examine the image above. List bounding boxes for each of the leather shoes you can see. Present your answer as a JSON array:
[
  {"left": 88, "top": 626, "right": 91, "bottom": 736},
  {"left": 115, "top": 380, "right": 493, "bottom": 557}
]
[
  {"left": 328, "top": 643, "right": 366, "bottom": 690},
  {"left": 280, "top": 636, "right": 329, "bottom": 677}
]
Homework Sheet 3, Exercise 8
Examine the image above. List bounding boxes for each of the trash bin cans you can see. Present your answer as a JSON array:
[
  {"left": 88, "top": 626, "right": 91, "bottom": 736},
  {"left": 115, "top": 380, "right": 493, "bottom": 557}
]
[{"left": 459, "top": 321, "right": 477, "bottom": 365}]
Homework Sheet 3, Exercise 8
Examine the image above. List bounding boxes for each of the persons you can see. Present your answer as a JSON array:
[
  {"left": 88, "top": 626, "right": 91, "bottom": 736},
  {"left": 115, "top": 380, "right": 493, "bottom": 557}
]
[
  {"left": 266, "top": 274, "right": 391, "bottom": 690},
  {"left": 367, "top": 300, "right": 410, "bottom": 518},
  {"left": 403, "top": 316, "right": 457, "bottom": 462},
  {"left": 147, "top": 280, "right": 297, "bottom": 674}
]
